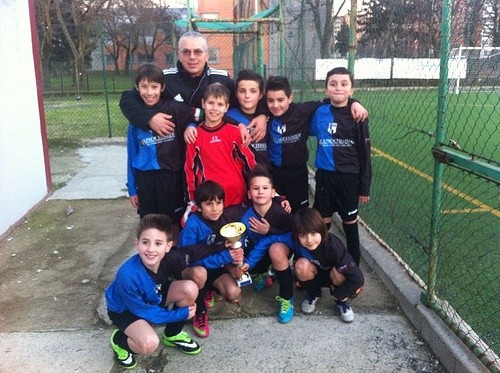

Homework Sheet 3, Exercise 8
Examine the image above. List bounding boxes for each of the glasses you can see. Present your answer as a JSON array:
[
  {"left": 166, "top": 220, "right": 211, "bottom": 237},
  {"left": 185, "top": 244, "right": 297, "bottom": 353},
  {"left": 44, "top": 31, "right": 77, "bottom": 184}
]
[{"left": 178, "top": 48, "right": 208, "bottom": 57}]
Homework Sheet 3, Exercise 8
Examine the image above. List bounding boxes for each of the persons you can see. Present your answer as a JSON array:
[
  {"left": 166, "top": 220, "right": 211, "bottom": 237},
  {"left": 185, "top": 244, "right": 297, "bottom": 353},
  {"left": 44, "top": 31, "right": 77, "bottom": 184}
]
[
  {"left": 105, "top": 33, "right": 372, "bottom": 369},
  {"left": 127, "top": 64, "right": 252, "bottom": 223}
]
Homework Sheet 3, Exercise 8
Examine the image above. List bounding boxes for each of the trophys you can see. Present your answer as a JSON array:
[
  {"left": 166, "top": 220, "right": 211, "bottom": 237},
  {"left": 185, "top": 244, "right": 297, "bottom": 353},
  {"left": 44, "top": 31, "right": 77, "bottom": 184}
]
[{"left": 220, "top": 222, "right": 252, "bottom": 287}]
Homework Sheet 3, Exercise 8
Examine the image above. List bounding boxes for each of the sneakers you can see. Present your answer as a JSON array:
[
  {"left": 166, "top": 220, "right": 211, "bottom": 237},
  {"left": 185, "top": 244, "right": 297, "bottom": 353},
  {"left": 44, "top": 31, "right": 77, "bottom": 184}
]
[
  {"left": 275, "top": 295, "right": 295, "bottom": 325},
  {"left": 191, "top": 301, "right": 210, "bottom": 337},
  {"left": 301, "top": 294, "right": 322, "bottom": 314},
  {"left": 160, "top": 329, "right": 202, "bottom": 355},
  {"left": 333, "top": 298, "right": 355, "bottom": 323},
  {"left": 204, "top": 291, "right": 216, "bottom": 308},
  {"left": 253, "top": 273, "right": 274, "bottom": 291},
  {"left": 109, "top": 329, "right": 138, "bottom": 370}
]
